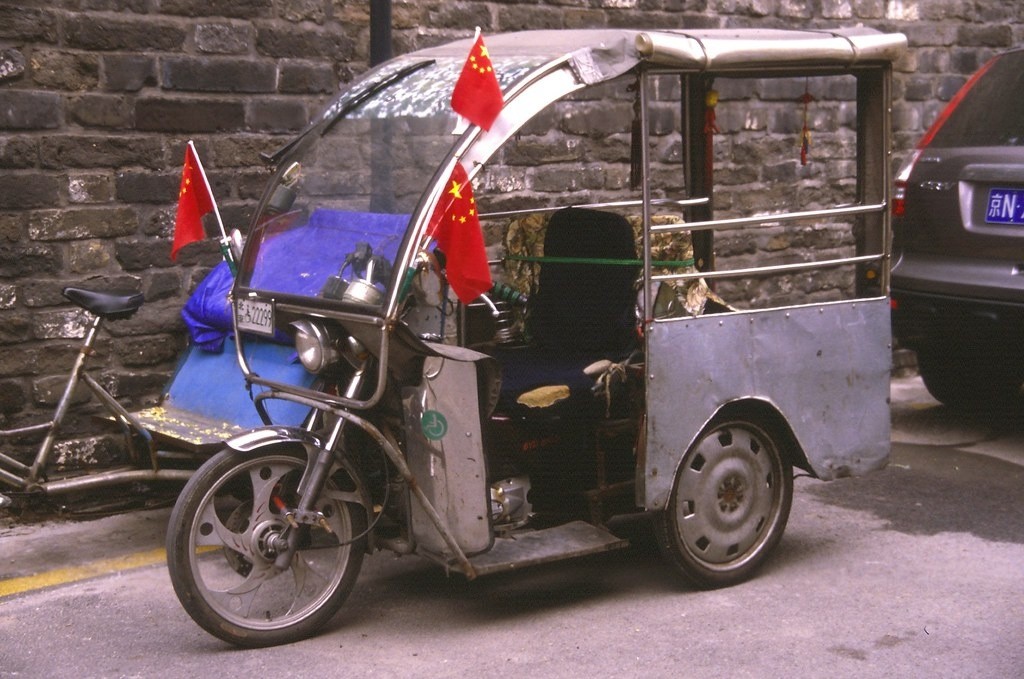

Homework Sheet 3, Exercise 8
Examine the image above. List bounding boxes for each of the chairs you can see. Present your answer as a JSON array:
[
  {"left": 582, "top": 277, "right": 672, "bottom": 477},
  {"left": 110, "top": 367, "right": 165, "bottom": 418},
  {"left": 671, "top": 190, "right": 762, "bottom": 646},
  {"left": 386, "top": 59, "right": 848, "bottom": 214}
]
[{"left": 472, "top": 208, "right": 639, "bottom": 416}]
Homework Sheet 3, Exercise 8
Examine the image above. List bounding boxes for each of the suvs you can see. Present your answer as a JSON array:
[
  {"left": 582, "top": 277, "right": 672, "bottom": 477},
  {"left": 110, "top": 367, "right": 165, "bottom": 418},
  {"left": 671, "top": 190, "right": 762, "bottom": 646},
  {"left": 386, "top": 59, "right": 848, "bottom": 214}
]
[{"left": 889, "top": 44, "right": 1024, "bottom": 413}]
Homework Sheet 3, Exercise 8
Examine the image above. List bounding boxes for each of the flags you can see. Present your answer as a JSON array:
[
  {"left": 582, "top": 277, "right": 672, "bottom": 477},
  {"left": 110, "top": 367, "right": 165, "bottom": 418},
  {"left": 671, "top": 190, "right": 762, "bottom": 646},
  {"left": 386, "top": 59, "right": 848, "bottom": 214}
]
[
  {"left": 172, "top": 144, "right": 212, "bottom": 261},
  {"left": 451, "top": 34, "right": 504, "bottom": 131},
  {"left": 425, "top": 162, "right": 492, "bottom": 305}
]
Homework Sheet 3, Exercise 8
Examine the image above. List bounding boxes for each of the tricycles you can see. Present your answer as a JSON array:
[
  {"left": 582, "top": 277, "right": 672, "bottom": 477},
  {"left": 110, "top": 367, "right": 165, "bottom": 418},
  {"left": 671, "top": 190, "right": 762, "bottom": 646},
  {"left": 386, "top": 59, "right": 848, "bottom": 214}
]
[
  {"left": 169, "top": 23, "right": 910, "bottom": 648},
  {"left": 2, "top": 211, "right": 437, "bottom": 525}
]
